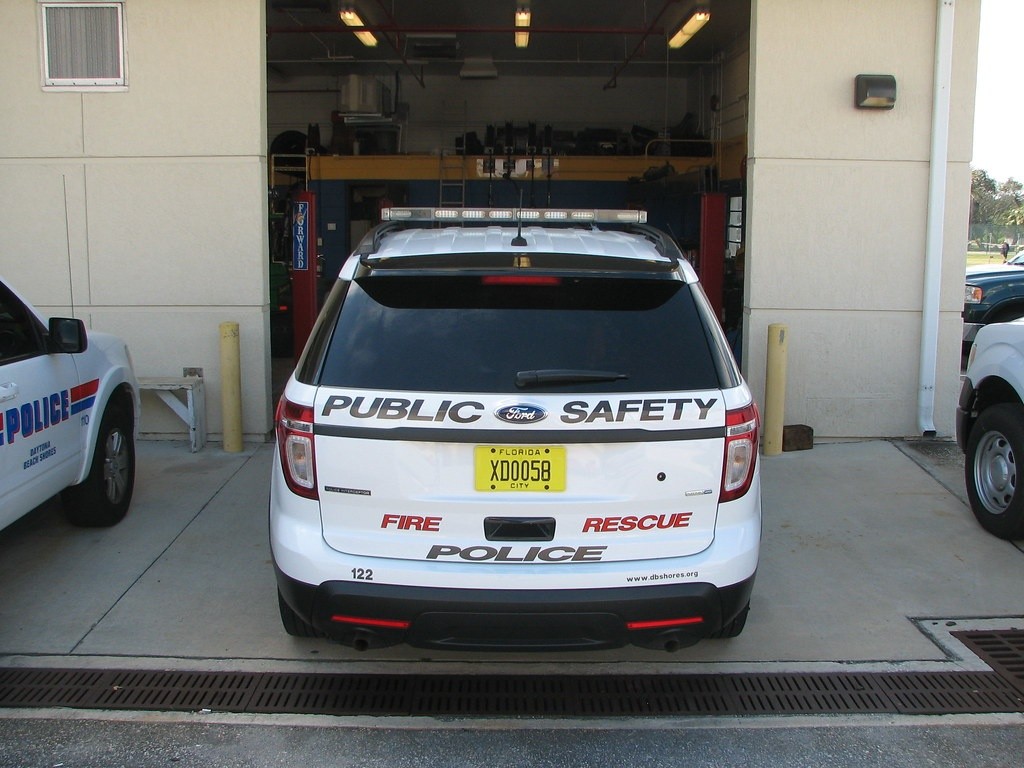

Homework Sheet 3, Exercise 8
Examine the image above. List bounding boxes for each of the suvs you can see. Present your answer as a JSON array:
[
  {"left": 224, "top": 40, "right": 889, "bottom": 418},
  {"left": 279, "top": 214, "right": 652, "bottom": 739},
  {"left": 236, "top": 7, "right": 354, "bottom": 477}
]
[{"left": 268, "top": 221, "right": 761, "bottom": 654}]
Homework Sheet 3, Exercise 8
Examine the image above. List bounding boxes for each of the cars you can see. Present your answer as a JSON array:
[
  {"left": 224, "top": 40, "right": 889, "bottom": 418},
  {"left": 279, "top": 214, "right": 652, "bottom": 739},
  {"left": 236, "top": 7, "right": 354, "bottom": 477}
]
[
  {"left": 961, "top": 250, "right": 1024, "bottom": 324},
  {"left": 0, "top": 276, "right": 142, "bottom": 533},
  {"left": 956, "top": 315, "right": 1024, "bottom": 540}
]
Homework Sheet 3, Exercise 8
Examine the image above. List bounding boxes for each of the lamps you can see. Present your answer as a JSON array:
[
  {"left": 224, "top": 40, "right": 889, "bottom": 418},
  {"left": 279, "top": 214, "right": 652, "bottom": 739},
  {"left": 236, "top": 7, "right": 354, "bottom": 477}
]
[
  {"left": 513, "top": 7, "right": 531, "bottom": 48},
  {"left": 669, "top": 8, "right": 711, "bottom": 50},
  {"left": 341, "top": 9, "right": 379, "bottom": 47}
]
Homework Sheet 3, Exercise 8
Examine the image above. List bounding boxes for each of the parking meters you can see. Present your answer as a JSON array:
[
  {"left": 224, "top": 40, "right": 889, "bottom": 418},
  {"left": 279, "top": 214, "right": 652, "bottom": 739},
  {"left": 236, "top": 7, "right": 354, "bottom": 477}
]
[{"left": 1003, "top": 242, "right": 1009, "bottom": 263}]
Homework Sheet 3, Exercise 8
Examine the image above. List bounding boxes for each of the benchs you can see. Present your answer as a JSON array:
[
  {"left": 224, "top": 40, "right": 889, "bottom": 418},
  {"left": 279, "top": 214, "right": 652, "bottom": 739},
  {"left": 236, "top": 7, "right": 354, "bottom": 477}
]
[{"left": 135, "top": 375, "right": 206, "bottom": 452}]
